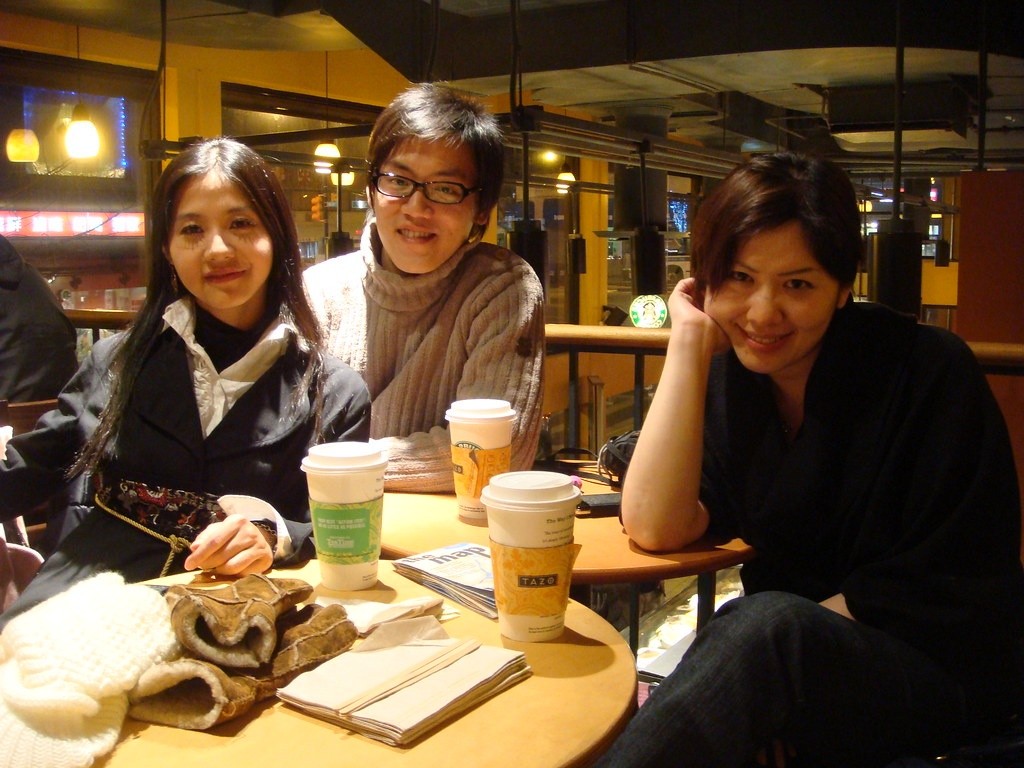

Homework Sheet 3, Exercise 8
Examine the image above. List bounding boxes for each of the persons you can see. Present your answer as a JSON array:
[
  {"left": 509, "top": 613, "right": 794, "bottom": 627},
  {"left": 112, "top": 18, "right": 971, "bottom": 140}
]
[
  {"left": 0, "top": 136, "right": 371, "bottom": 637},
  {"left": 591, "top": 150, "right": 1024, "bottom": 768},
  {"left": 303, "top": 82, "right": 545, "bottom": 495}
]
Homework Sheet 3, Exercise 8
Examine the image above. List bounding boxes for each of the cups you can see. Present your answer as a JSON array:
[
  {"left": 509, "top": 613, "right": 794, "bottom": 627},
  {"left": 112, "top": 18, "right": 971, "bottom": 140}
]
[
  {"left": 481, "top": 471, "right": 580, "bottom": 641},
  {"left": 299, "top": 442, "right": 388, "bottom": 591},
  {"left": 445, "top": 399, "right": 516, "bottom": 519}
]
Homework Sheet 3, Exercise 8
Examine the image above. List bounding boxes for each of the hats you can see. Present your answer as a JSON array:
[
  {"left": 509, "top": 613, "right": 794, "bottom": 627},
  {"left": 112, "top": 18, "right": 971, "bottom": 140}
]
[{"left": 0, "top": 571, "right": 182, "bottom": 768}]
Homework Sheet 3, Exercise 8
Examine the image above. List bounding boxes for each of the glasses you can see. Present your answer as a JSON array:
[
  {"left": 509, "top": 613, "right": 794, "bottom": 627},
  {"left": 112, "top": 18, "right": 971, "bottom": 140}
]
[{"left": 372, "top": 166, "right": 483, "bottom": 204}]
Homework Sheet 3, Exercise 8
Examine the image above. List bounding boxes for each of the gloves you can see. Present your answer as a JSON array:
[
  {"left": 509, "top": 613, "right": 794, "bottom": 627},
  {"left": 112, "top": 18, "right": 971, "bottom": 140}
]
[
  {"left": 157, "top": 569, "right": 313, "bottom": 668},
  {"left": 127, "top": 603, "right": 359, "bottom": 729}
]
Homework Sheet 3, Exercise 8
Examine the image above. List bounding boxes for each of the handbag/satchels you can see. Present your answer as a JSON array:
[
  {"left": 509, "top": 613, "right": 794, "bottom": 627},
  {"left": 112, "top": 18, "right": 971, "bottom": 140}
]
[
  {"left": 0, "top": 478, "right": 227, "bottom": 632},
  {"left": 598, "top": 428, "right": 642, "bottom": 487}
]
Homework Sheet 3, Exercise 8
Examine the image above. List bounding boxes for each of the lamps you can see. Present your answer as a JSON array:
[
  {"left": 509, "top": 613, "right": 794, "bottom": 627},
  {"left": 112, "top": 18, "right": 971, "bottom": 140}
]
[
  {"left": 315, "top": 50, "right": 341, "bottom": 171},
  {"left": 64, "top": 25, "right": 102, "bottom": 160},
  {"left": 5, "top": 121, "right": 42, "bottom": 162},
  {"left": 556, "top": 161, "right": 578, "bottom": 193},
  {"left": 332, "top": 163, "right": 356, "bottom": 188}
]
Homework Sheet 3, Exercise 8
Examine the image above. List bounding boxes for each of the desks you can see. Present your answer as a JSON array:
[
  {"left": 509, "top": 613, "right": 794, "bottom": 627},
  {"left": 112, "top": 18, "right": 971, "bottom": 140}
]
[
  {"left": 381, "top": 440, "right": 758, "bottom": 715},
  {"left": 0, "top": 554, "right": 636, "bottom": 766}
]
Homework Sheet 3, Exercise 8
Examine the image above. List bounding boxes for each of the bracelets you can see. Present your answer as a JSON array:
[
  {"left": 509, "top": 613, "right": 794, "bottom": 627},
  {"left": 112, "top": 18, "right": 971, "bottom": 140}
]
[{"left": 250, "top": 521, "right": 277, "bottom": 537}]
